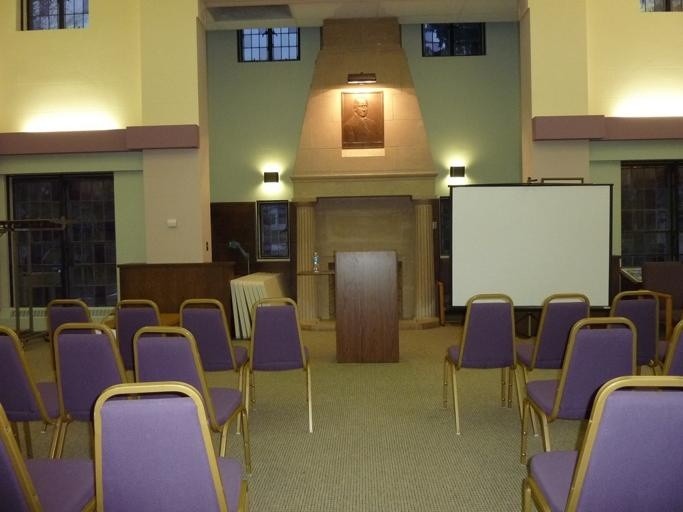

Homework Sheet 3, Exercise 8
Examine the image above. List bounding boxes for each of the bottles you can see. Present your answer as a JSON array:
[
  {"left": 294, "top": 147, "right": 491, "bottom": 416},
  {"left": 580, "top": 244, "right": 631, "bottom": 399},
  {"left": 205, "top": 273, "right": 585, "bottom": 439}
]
[{"left": 311, "top": 250, "right": 320, "bottom": 273}]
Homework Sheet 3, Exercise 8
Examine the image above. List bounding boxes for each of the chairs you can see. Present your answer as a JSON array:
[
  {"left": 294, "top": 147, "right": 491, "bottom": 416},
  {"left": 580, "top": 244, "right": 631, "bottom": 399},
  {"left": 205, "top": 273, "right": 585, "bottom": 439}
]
[
  {"left": 0, "top": 324, "right": 61, "bottom": 458},
  {"left": 245, "top": 296, "right": 313, "bottom": 433},
  {"left": 506, "top": 293, "right": 590, "bottom": 437},
  {"left": 522, "top": 375, "right": 683, "bottom": 511},
  {"left": 46, "top": 300, "right": 97, "bottom": 383},
  {"left": 520, "top": 317, "right": 637, "bottom": 465},
  {"left": 93, "top": 380, "right": 246, "bottom": 510},
  {"left": 638, "top": 318, "right": 683, "bottom": 376},
  {"left": 53, "top": 323, "right": 129, "bottom": 458},
  {"left": 0, "top": 399, "right": 96, "bottom": 510},
  {"left": 116, "top": 299, "right": 162, "bottom": 383},
  {"left": 179, "top": 299, "right": 256, "bottom": 403},
  {"left": 133, "top": 326, "right": 252, "bottom": 466},
  {"left": 608, "top": 290, "right": 659, "bottom": 375},
  {"left": 443, "top": 293, "right": 528, "bottom": 435}
]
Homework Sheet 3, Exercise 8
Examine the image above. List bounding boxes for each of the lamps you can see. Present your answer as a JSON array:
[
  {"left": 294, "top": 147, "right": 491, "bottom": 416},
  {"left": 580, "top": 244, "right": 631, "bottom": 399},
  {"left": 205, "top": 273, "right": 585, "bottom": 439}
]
[
  {"left": 450, "top": 167, "right": 465, "bottom": 177},
  {"left": 347, "top": 72, "right": 376, "bottom": 83},
  {"left": 264, "top": 172, "right": 278, "bottom": 182}
]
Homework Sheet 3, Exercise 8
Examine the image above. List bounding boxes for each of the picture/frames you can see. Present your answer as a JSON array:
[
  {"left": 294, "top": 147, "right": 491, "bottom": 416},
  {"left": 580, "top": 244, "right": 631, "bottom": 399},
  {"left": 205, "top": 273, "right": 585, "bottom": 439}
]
[{"left": 255, "top": 200, "right": 290, "bottom": 259}]
